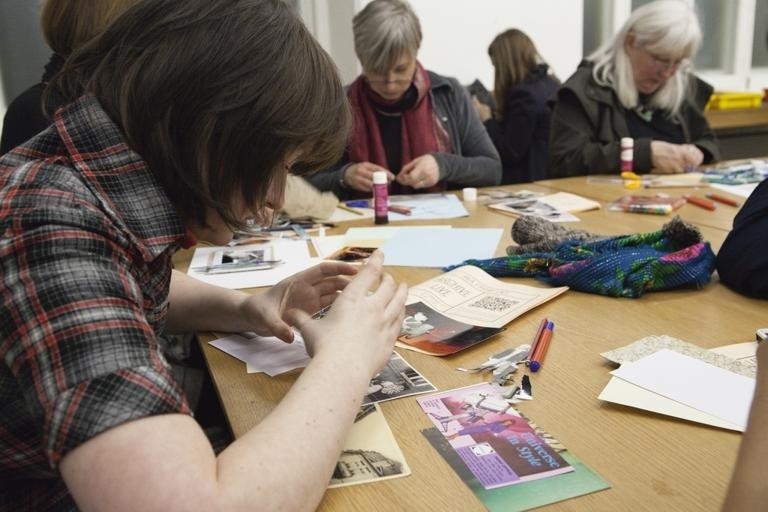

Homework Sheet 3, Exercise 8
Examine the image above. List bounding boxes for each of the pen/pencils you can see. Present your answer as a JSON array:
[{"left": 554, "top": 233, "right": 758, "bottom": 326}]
[
  {"left": 622, "top": 194, "right": 686, "bottom": 215},
  {"left": 260, "top": 224, "right": 322, "bottom": 232},
  {"left": 388, "top": 206, "right": 411, "bottom": 216},
  {"left": 335, "top": 204, "right": 364, "bottom": 216},
  {"left": 686, "top": 190, "right": 740, "bottom": 212},
  {"left": 644, "top": 179, "right": 663, "bottom": 190},
  {"left": 526, "top": 318, "right": 555, "bottom": 372}
]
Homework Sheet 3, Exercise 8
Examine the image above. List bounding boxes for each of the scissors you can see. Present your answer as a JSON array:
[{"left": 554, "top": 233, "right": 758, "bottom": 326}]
[
  {"left": 346, "top": 200, "right": 375, "bottom": 210},
  {"left": 621, "top": 170, "right": 644, "bottom": 191}
]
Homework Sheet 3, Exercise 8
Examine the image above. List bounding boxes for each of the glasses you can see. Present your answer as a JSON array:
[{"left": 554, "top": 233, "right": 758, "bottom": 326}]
[{"left": 366, "top": 80, "right": 411, "bottom": 86}]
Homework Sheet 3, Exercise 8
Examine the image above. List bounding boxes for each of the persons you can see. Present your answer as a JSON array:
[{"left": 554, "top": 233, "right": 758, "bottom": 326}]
[
  {"left": 304, "top": 1, "right": 504, "bottom": 201},
  {"left": 713, "top": 336, "right": 768, "bottom": 511},
  {"left": 715, "top": 176, "right": 768, "bottom": 302},
  {"left": 1, "top": 0, "right": 407, "bottom": 512},
  {"left": 540, "top": 1, "right": 720, "bottom": 179},
  {"left": 470, "top": 29, "right": 563, "bottom": 183},
  {"left": 1, "top": 0, "right": 134, "bottom": 156}
]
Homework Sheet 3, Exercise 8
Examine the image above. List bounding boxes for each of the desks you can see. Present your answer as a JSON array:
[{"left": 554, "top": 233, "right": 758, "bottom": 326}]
[
  {"left": 168, "top": 156, "right": 768, "bottom": 511},
  {"left": 701, "top": 107, "right": 768, "bottom": 137}
]
